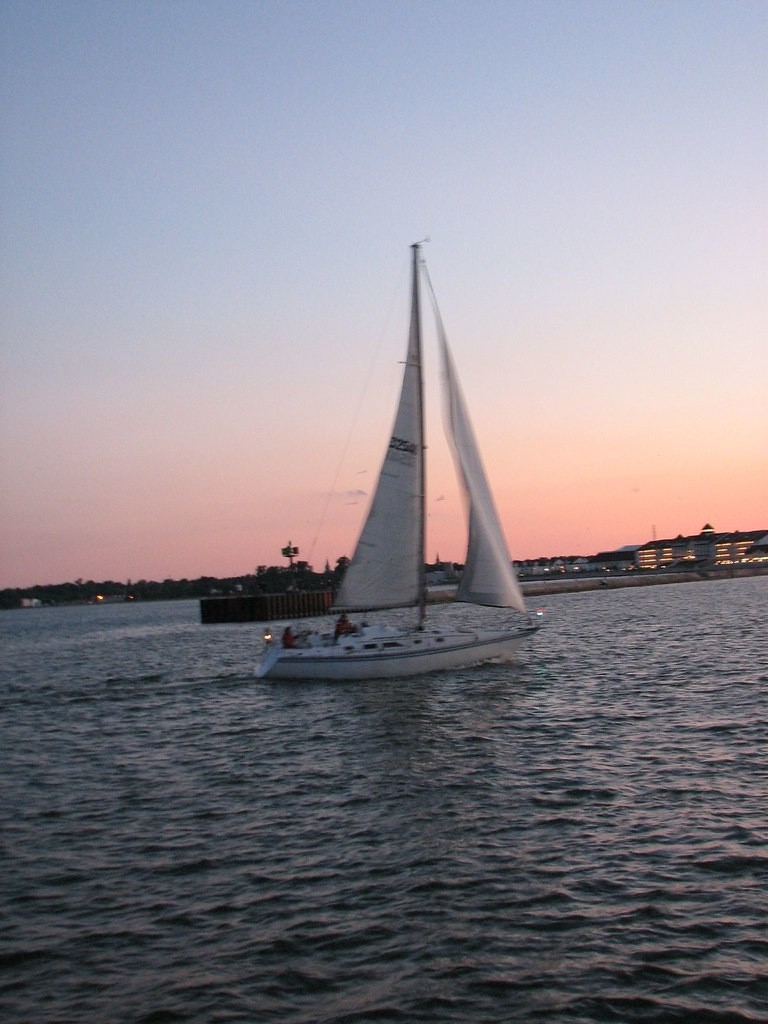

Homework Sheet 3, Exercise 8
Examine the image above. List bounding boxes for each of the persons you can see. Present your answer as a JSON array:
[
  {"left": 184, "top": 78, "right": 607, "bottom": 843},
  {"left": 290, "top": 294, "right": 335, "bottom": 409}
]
[
  {"left": 282, "top": 626, "right": 301, "bottom": 648},
  {"left": 334, "top": 614, "right": 352, "bottom": 640}
]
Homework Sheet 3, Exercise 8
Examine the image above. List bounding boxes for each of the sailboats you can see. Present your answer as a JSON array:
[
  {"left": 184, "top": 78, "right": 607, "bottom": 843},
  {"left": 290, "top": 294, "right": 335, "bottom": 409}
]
[{"left": 251, "top": 244, "right": 541, "bottom": 680}]
